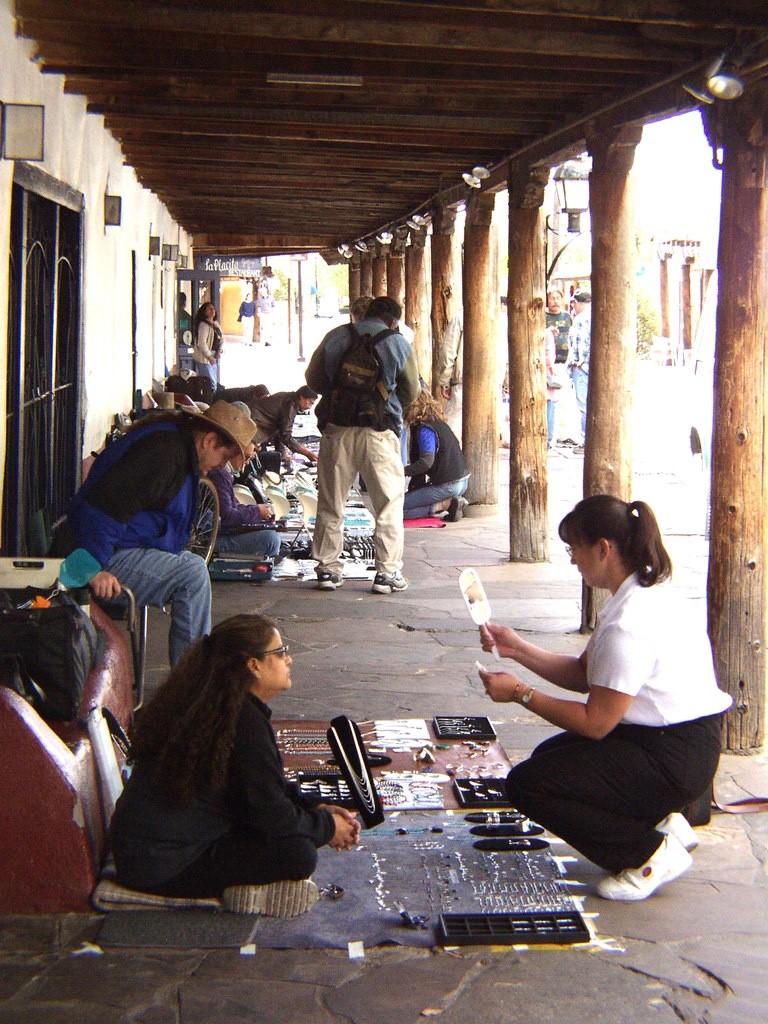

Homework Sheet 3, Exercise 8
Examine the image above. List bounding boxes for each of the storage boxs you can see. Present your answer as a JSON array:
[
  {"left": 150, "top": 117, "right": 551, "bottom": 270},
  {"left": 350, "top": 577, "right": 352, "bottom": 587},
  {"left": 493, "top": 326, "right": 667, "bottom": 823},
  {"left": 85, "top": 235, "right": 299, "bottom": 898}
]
[{"left": 209, "top": 555, "right": 275, "bottom": 584}]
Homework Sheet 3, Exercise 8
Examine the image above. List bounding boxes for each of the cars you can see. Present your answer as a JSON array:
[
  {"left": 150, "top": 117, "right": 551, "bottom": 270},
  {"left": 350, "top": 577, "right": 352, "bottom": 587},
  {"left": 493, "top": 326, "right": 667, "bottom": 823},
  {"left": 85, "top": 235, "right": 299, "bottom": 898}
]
[{"left": 689, "top": 262, "right": 720, "bottom": 492}]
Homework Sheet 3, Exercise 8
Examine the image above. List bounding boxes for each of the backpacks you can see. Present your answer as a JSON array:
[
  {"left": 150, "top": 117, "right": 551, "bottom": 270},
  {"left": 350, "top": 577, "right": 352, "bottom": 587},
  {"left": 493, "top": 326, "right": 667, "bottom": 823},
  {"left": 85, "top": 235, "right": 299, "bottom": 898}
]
[{"left": 328, "top": 322, "right": 404, "bottom": 427}]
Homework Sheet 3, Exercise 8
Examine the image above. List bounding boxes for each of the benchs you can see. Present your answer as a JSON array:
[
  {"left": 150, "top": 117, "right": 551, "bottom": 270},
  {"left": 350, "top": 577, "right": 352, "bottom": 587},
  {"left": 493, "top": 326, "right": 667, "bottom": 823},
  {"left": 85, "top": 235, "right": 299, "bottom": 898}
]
[{"left": 0, "top": 579, "right": 132, "bottom": 915}]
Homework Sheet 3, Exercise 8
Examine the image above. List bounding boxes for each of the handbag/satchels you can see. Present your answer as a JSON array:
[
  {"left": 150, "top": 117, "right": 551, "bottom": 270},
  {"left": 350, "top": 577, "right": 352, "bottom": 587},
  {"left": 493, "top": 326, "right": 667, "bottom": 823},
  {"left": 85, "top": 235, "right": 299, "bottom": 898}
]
[{"left": 0, "top": 586, "right": 106, "bottom": 723}]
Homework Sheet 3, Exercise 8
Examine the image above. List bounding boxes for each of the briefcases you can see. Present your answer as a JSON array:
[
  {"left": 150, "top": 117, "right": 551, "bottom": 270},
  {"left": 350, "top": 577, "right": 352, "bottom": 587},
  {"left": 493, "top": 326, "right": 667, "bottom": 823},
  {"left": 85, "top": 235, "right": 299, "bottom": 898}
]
[{"left": 206, "top": 556, "right": 273, "bottom": 582}]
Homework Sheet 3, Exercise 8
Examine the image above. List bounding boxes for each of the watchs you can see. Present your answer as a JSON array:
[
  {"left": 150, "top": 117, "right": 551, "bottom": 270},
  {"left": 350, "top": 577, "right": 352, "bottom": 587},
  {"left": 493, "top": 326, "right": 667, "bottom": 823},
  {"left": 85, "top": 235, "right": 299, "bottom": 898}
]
[{"left": 520, "top": 687, "right": 535, "bottom": 706}]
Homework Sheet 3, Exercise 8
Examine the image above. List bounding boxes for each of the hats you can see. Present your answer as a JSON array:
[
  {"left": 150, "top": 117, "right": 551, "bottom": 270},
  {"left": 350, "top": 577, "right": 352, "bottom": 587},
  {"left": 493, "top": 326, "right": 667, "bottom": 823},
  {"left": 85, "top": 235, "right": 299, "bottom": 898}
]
[
  {"left": 574, "top": 293, "right": 591, "bottom": 303},
  {"left": 182, "top": 400, "right": 257, "bottom": 473}
]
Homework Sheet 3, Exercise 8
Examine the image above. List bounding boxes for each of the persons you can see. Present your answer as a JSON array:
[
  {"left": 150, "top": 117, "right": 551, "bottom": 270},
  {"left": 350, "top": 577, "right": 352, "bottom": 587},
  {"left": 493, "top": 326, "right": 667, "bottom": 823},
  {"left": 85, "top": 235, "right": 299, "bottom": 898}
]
[
  {"left": 195, "top": 302, "right": 223, "bottom": 395},
  {"left": 239, "top": 293, "right": 255, "bottom": 346},
  {"left": 108, "top": 613, "right": 361, "bottom": 919},
  {"left": 195, "top": 384, "right": 317, "bottom": 567},
  {"left": 46, "top": 400, "right": 257, "bottom": 673},
  {"left": 478, "top": 495, "right": 733, "bottom": 900},
  {"left": 403, "top": 387, "right": 471, "bottom": 522},
  {"left": 545, "top": 286, "right": 575, "bottom": 445},
  {"left": 557, "top": 288, "right": 591, "bottom": 454},
  {"left": 303, "top": 297, "right": 423, "bottom": 596},
  {"left": 545, "top": 327, "right": 558, "bottom": 451},
  {"left": 258, "top": 287, "right": 276, "bottom": 346}
]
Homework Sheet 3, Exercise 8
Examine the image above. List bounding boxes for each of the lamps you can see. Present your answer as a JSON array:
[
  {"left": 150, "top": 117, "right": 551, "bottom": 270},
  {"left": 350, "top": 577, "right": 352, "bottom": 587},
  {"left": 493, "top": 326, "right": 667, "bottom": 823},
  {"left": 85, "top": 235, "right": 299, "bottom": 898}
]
[
  {"left": 546, "top": 160, "right": 591, "bottom": 302},
  {"left": 336, "top": 242, "right": 355, "bottom": 258},
  {"left": 376, "top": 225, "right": 397, "bottom": 247},
  {"left": 354, "top": 237, "right": 372, "bottom": 252},
  {"left": 406, "top": 210, "right": 433, "bottom": 231},
  {"left": 462, "top": 162, "right": 499, "bottom": 192},
  {"left": 682, "top": 46, "right": 752, "bottom": 106}
]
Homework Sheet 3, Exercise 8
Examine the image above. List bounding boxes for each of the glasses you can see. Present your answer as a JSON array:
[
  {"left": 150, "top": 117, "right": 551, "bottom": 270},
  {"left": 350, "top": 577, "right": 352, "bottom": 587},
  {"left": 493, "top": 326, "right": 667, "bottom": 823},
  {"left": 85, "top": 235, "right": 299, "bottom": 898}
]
[
  {"left": 566, "top": 534, "right": 608, "bottom": 555},
  {"left": 244, "top": 454, "right": 250, "bottom": 461},
  {"left": 256, "top": 645, "right": 288, "bottom": 658}
]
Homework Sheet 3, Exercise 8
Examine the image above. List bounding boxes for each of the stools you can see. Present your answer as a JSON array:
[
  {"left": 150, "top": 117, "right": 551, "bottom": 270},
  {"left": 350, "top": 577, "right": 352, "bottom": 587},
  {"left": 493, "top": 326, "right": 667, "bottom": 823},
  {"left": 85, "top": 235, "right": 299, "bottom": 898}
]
[{"left": 122, "top": 580, "right": 151, "bottom": 713}]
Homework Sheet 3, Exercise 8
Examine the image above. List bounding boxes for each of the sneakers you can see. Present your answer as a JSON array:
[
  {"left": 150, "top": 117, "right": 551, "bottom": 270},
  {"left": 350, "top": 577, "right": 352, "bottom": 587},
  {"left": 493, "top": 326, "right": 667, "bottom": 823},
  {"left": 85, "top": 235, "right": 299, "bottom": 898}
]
[
  {"left": 556, "top": 437, "right": 584, "bottom": 454},
  {"left": 317, "top": 570, "right": 343, "bottom": 590},
  {"left": 443, "top": 496, "right": 469, "bottom": 522},
  {"left": 654, "top": 812, "right": 699, "bottom": 854},
  {"left": 596, "top": 833, "right": 693, "bottom": 901},
  {"left": 222, "top": 879, "right": 317, "bottom": 916},
  {"left": 371, "top": 570, "right": 408, "bottom": 594}
]
